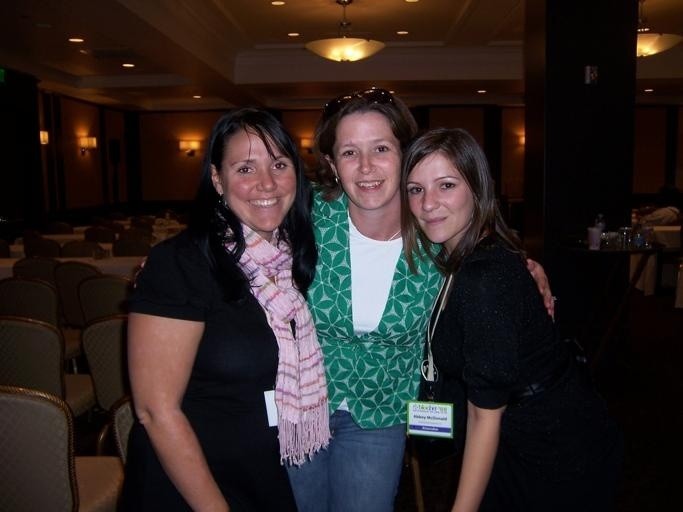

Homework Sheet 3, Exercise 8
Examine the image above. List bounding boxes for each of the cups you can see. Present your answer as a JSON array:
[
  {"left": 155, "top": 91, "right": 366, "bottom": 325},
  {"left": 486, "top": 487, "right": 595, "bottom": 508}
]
[
  {"left": 620, "top": 226, "right": 633, "bottom": 249},
  {"left": 588, "top": 227, "right": 600, "bottom": 250}
]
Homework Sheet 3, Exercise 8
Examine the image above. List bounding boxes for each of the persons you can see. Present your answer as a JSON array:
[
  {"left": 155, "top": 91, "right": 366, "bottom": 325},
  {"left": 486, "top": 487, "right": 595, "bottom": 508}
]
[
  {"left": 635, "top": 185, "right": 681, "bottom": 227},
  {"left": 113, "top": 107, "right": 315, "bottom": 511},
  {"left": 131, "top": 86, "right": 562, "bottom": 511},
  {"left": 398, "top": 126, "right": 626, "bottom": 511}
]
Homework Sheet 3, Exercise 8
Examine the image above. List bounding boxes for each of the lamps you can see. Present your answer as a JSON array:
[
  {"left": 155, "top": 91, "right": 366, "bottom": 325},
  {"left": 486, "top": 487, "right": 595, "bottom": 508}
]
[
  {"left": 300, "top": 137, "right": 312, "bottom": 155},
  {"left": 78, "top": 137, "right": 96, "bottom": 155},
  {"left": 179, "top": 141, "right": 198, "bottom": 157},
  {"left": 634, "top": 1, "right": 683, "bottom": 59},
  {"left": 301, "top": 0, "right": 385, "bottom": 63}
]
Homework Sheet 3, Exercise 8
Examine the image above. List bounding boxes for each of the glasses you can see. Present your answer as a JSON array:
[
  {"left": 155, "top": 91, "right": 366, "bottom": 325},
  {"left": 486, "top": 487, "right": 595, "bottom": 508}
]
[{"left": 322, "top": 88, "right": 390, "bottom": 105}]
